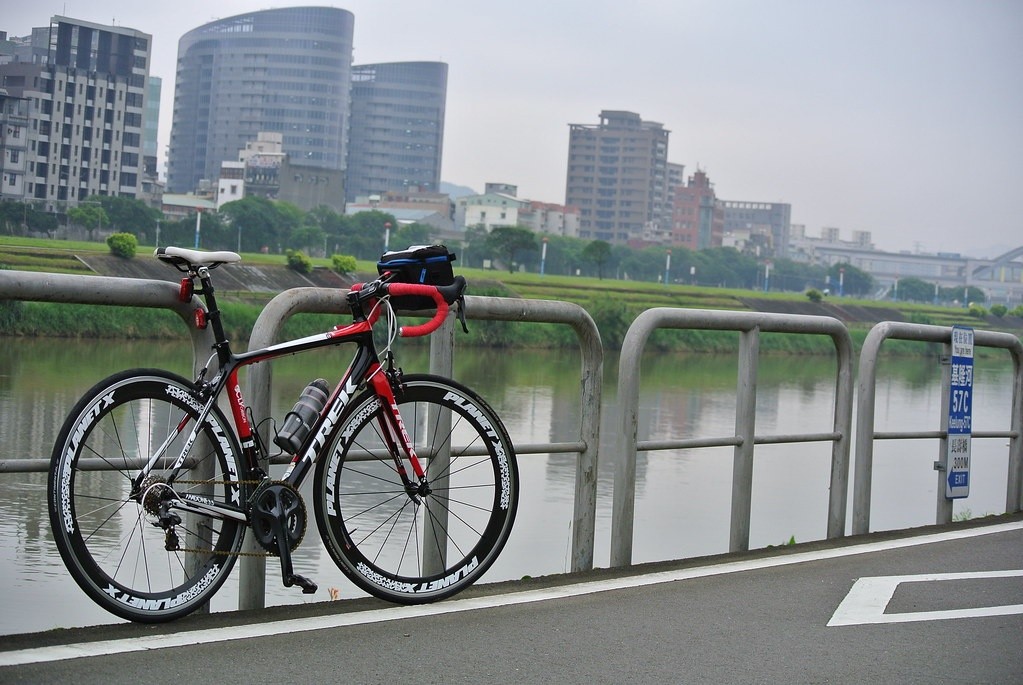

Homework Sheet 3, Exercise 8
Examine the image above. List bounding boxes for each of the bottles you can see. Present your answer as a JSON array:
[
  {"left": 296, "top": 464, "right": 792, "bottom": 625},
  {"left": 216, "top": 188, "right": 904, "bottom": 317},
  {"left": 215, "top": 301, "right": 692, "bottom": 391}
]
[{"left": 273, "top": 378, "right": 331, "bottom": 454}]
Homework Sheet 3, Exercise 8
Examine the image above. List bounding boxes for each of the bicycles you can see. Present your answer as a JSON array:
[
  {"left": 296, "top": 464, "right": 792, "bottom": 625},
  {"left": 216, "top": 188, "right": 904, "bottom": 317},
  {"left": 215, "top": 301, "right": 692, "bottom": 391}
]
[{"left": 46, "top": 246, "right": 519, "bottom": 621}]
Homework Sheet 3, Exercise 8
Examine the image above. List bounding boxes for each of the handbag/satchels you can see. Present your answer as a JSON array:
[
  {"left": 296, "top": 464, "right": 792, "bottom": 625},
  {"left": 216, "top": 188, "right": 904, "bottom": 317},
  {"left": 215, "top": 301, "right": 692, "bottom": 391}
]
[{"left": 377, "top": 244, "right": 456, "bottom": 310}]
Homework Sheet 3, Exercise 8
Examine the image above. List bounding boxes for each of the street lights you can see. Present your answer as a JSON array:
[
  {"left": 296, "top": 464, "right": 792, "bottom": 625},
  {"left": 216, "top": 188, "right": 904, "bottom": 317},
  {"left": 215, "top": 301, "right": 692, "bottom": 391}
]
[
  {"left": 194, "top": 205, "right": 204, "bottom": 247},
  {"left": 763, "top": 259, "right": 771, "bottom": 291},
  {"left": 894, "top": 275, "right": 900, "bottom": 302},
  {"left": 384, "top": 223, "right": 390, "bottom": 254},
  {"left": 663, "top": 249, "right": 672, "bottom": 285},
  {"left": 839, "top": 268, "right": 845, "bottom": 298},
  {"left": 541, "top": 237, "right": 548, "bottom": 277}
]
